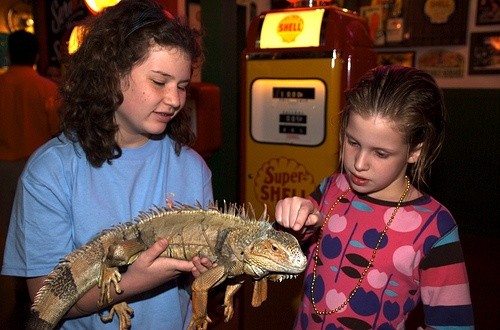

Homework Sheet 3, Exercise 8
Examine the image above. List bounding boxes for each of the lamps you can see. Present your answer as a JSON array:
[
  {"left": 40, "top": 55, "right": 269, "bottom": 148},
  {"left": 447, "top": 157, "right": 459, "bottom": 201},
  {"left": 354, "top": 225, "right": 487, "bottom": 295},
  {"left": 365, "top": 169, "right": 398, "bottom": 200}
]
[
  {"left": 66, "top": 24, "right": 87, "bottom": 56},
  {"left": 83, "top": 0, "right": 123, "bottom": 14}
]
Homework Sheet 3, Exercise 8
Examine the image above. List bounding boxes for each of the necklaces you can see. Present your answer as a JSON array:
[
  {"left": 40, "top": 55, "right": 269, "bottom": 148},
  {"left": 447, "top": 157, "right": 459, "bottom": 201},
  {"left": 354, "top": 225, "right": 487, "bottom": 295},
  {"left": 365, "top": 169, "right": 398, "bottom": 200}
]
[{"left": 311, "top": 176, "right": 410, "bottom": 314}]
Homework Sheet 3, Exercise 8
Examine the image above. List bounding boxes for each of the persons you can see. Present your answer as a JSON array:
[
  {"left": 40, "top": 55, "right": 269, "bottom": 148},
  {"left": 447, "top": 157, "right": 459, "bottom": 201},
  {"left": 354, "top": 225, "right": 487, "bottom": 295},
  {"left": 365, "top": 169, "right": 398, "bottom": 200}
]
[
  {"left": 0, "top": 1, "right": 219, "bottom": 330},
  {"left": 275, "top": 65, "right": 475, "bottom": 330}
]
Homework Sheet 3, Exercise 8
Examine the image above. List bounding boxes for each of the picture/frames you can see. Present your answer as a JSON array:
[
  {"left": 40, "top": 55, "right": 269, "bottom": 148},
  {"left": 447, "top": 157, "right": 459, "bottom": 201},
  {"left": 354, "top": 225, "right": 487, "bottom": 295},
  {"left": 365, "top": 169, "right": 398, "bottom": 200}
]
[
  {"left": 475, "top": 0, "right": 500, "bottom": 26},
  {"left": 380, "top": 49, "right": 415, "bottom": 68},
  {"left": 467, "top": 30, "right": 500, "bottom": 74}
]
